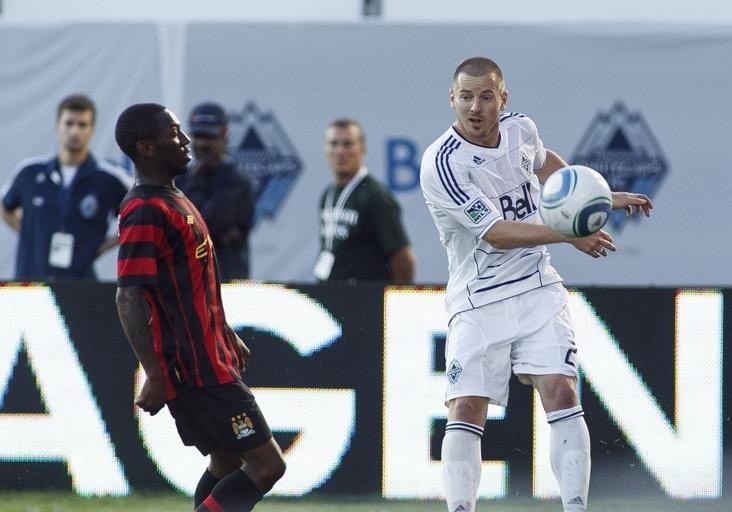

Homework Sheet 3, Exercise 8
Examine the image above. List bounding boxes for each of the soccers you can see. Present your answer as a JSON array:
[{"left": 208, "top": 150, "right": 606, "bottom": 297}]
[{"left": 538, "top": 164, "right": 613, "bottom": 237}]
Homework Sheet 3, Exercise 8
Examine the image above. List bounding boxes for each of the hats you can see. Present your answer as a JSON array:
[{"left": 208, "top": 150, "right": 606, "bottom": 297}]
[{"left": 187, "top": 104, "right": 226, "bottom": 138}]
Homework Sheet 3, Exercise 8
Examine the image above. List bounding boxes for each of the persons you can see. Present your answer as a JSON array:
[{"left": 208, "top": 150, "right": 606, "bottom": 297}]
[
  {"left": 178, "top": 105, "right": 256, "bottom": 280},
  {"left": 111, "top": 100, "right": 287, "bottom": 511},
  {"left": 310, "top": 117, "right": 418, "bottom": 281},
  {"left": 418, "top": 53, "right": 656, "bottom": 511},
  {"left": 0, "top": 93, "right": 133, "bottom": 281}
]
